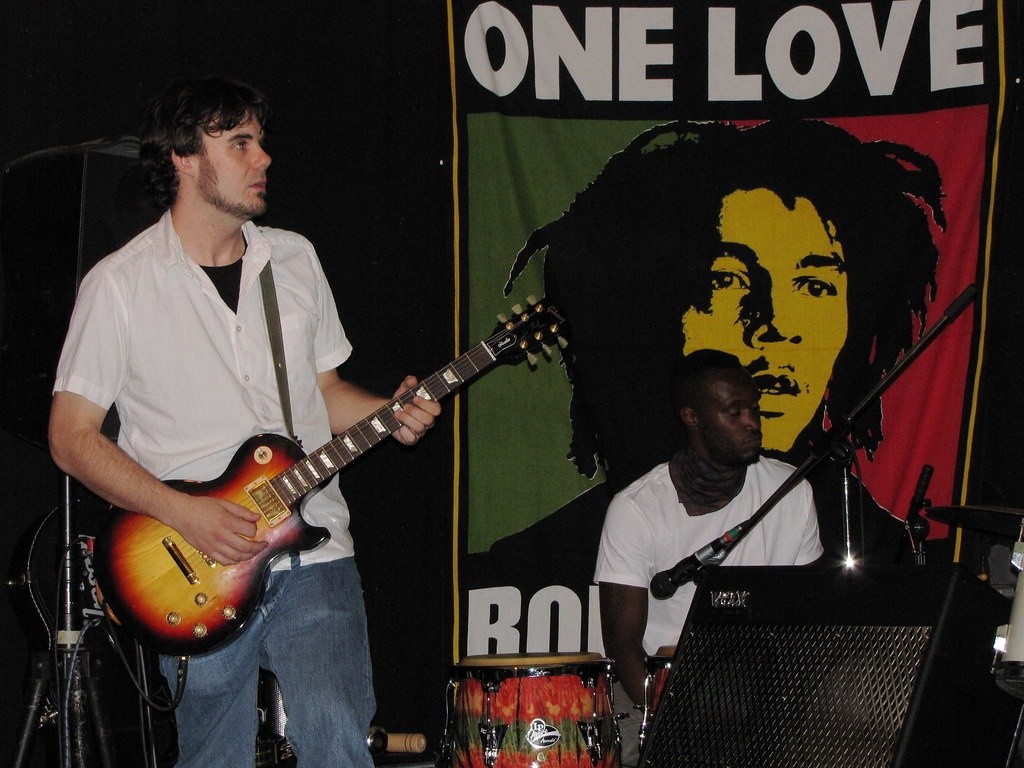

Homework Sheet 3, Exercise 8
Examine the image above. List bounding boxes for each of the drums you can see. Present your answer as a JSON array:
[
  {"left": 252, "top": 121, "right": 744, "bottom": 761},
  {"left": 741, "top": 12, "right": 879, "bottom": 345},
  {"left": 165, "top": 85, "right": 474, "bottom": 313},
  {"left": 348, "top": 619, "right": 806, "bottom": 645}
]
[
  {"left": 638, "top": 645, "right": 679, "bottom": 753},
  {"left": 440, "top": 651, "right": 620, "bottom": 768}
]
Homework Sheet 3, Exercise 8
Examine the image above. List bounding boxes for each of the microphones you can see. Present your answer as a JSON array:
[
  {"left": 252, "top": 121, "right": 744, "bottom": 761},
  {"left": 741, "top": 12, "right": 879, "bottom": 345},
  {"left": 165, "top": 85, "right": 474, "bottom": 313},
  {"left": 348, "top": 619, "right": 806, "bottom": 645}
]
[{"left": 650, "top": 524, "right": 748, "bottom": 600}]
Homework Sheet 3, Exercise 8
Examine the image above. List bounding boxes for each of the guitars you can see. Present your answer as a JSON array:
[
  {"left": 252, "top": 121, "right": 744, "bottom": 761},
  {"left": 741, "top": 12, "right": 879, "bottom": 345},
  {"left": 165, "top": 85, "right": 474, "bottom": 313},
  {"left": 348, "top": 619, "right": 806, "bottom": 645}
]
[{"left": 91, "top": 295, "right": 571, "bottom": 658}]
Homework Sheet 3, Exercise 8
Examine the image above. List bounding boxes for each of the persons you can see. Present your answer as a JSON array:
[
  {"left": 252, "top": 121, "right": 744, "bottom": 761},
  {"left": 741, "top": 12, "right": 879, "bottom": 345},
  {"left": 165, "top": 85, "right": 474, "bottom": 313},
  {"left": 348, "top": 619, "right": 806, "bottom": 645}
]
[
  {"left": 48, "top": 70, "right": 443, "bottom": 768},
  {"left": 593, "top": 348, "right": 824, "bottom": 768}
]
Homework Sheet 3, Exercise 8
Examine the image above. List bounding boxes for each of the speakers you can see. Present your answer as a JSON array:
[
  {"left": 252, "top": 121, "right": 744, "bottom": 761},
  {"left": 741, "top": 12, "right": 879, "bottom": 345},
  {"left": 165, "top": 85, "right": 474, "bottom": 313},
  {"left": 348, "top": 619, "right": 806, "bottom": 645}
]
[
  {"left": 0, "top": 134, "right": 165, "bottom": 450},
  {"left": 640, "top": 562, "right": 1024, "bottom": 768}
]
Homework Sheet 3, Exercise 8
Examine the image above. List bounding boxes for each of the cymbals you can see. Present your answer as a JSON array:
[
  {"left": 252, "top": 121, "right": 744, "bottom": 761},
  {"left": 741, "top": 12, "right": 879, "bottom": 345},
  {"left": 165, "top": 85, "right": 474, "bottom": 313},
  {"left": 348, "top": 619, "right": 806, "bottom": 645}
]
[{"left": 923, "top": 504, "right": 1024, "bottom": 531}]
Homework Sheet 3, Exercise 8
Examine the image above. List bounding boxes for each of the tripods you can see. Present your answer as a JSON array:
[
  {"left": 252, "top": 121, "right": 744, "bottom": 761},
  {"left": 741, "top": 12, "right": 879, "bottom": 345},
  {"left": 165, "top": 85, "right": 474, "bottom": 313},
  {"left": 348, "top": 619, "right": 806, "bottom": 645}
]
[{"left": 13, "top": 474, "right": 113, "bottom": 768}]
